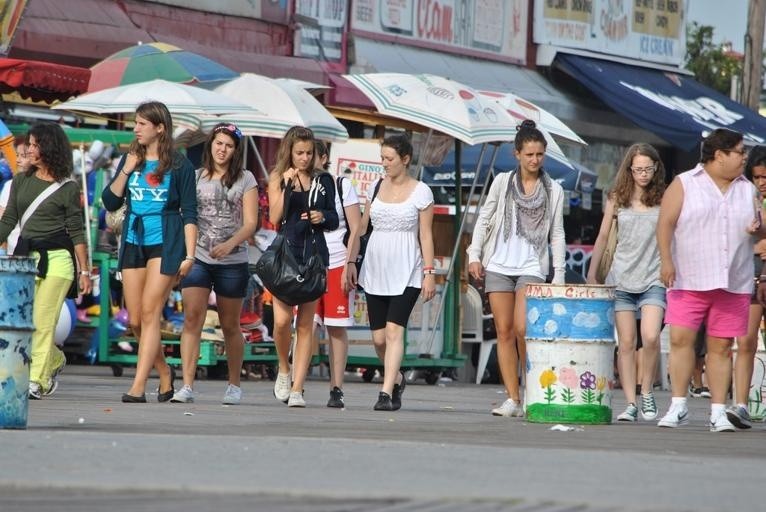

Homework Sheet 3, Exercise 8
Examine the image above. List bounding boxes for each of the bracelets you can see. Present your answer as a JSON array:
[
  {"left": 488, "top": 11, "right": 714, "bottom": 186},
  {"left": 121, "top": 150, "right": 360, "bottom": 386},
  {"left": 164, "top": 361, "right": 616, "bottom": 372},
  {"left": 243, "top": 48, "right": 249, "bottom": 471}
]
[{"left": 121, "top": 167, "right": 130, "bottom": 178}]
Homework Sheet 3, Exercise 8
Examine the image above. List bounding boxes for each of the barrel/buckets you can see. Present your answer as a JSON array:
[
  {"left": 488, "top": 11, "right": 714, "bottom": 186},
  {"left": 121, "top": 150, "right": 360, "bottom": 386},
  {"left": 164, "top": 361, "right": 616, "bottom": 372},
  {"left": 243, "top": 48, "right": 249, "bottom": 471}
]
[
  {"left": 731, "top": 328, "right": 765, "bottom": 422},
  {"left": 0, "top": 255, "right": 39, "bottom": 429},
  {"left": 524, "top": 283, "right": 620, "bottom": 423}
]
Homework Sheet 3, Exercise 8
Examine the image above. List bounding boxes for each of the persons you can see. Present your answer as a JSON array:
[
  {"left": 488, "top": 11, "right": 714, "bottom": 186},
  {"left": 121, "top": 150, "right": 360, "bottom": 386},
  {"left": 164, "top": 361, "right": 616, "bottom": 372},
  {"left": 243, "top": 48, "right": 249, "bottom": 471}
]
[
  {"left": 587, "top": 129, "right": 766, "bottom": 434},
  {"left": 467, "top": 120, "right": 567, "bottom": 415},
  {"left": 0, "top": 100, "right": 437, "bottom": 412}
]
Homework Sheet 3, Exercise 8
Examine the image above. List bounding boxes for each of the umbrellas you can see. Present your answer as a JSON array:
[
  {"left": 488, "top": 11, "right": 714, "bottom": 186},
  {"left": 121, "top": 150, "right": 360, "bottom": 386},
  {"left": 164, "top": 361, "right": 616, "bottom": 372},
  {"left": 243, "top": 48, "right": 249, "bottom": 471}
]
[
  {"left": 466, "top": 90, "right": 587, "bottom": 248},
  {"left": 169, "top": 72, "right": 349, "bottom": 145},
  {"left": 339, "top": 69, "right": 521, "bottom": 180},
  {"left": 50, "top": 78, "right": 268, "bottom": 131},
  {"left": 83, "top": 40, "right": 239, "bottom": 93}
]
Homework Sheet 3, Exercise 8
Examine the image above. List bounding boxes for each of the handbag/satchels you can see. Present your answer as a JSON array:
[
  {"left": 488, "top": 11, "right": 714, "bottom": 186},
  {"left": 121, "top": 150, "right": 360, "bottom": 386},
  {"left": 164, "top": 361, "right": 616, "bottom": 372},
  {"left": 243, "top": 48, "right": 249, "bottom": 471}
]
[
  {"left": 465, "top": 213, "right": 496, "bottom": 259},
  {"left": 254, "top": 232, "right": 327, "bottom": 305},
  {"left": 337, "top": 176, "right": 373, "bottom": 256},
  {"left": 592, "top": 200, "right": 620, "bottom": 284}
]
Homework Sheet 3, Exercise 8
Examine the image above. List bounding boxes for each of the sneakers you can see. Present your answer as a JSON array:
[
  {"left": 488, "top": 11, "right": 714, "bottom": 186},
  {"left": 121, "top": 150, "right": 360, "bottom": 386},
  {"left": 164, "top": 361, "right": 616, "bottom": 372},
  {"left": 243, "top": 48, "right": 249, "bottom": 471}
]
[
  {"left": 615, "top": 402, "right": 637, "bottom": 422},
  {"left": 490, "top": 397, "right": 523, "bottom": 418},
  {"left": 328, "top": 387, "right": 345, "bottom": 408},
  {"left": 689, "top": 386, "right": 711, "bottom": 399},
  {"left": 171, "top": 385, "right": 194, "bottom": 403},
  {"left": 657, "top": 404, "right": 691, "bottom": 428},
  {"left": 641, "top": 390, "right": 658, "bottom": 420},
  {"left": 374, "top": 372, "right": 406, "bottom": 411},
  {"left": 708, "top": 406, "right": 751, "bottom": 433},
  {"left": 273, "top": 366, "right": 306, "bottom": 407},
  {"left": 222, "top": 383, "right": 242, "bottom": 405},
  {"left": 29, "top": 350, "right": 67, "bottom": 400}
]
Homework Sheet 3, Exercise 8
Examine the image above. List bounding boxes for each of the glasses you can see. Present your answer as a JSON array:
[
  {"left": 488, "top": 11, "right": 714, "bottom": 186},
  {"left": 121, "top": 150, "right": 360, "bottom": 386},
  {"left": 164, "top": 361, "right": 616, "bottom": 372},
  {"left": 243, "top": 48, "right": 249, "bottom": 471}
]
[
  {"left": 717, "top": 146, "right": 747, "bottom": 155},
  {"left": 627, "top": 166, "right": 656, "bottom": 174}
]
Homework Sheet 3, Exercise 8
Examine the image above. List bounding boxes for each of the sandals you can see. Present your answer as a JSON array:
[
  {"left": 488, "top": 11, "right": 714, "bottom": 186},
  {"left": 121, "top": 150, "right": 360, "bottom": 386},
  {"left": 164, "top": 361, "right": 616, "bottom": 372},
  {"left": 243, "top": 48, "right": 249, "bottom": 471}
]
[
  {"left": 121, "top": 393, "right": 148, "bottom": 403},
  {"left": 158, "top": 363, "right": 176, "bottom": 401}
]
